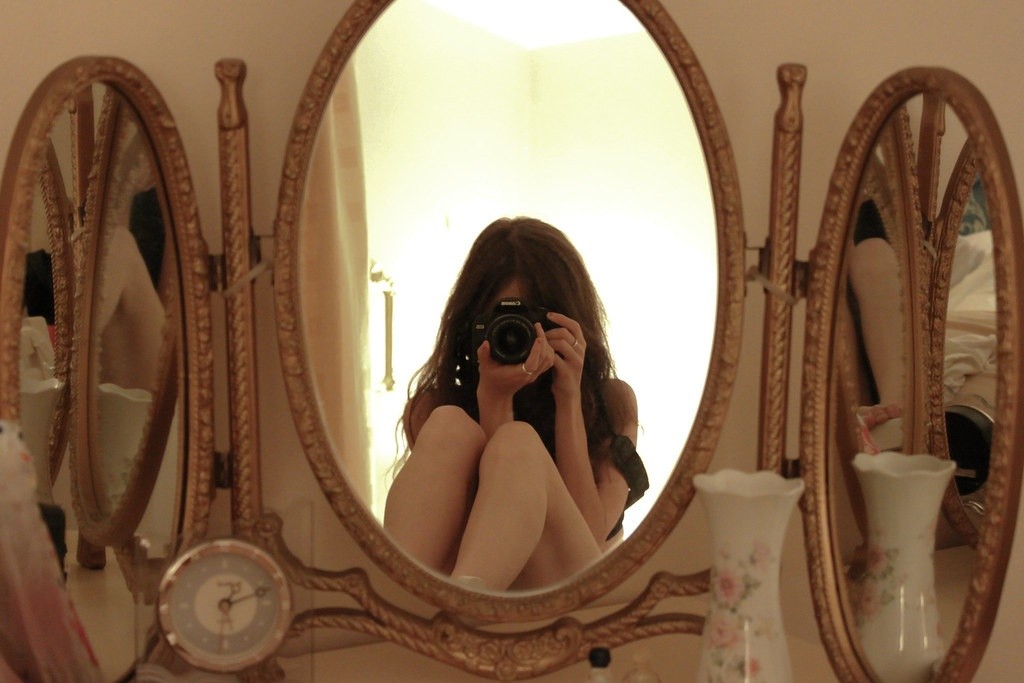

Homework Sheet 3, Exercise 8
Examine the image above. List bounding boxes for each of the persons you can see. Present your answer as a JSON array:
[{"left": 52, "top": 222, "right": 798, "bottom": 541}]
[
  {"left": 383, "top": 218, "right": 639, "bottom": 595},
  {"left": 96, "top": 164, "right": 168, "bottom": 392}
]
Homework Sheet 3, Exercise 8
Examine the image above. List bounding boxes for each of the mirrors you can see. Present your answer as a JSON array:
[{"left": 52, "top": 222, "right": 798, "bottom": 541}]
[
  {"left": 277, "top": 0, "right": 748, "bottom": 618},
  {"left": 18, "top": 140, "right": 65, "bottom": 490},
  {"left": 1, "top": 56, "right": 218, "bottom": 683},
  {"left": 833, "top": 98, "right": 933, "bottom": 545},
  {"left": 68, "top": 84, "right": 185, "bottom": 547},
  {"left": 929, "top": 129, "right": 997, "bottom": 548},
  {"left": 802, "top": 62, "right": 1022, "bottom": 683}
]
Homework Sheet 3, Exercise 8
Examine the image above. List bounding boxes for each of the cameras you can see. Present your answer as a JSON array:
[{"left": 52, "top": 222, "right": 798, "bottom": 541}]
[{"left": 473, "top": 297, "right": 566, "bottom": 368}]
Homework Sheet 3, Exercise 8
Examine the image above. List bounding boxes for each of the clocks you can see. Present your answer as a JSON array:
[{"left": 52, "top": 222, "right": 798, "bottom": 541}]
[{"left": 161, "top": 539, "right": 295, "bottom": 674}]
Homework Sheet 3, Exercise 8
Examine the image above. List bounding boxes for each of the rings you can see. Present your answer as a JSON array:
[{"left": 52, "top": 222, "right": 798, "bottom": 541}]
[
  {"left": 522, "top": 364, "right": 532, "bottom": 375},
  {"left": 573, "top": 340, "right": 578, "bottom": 348}
]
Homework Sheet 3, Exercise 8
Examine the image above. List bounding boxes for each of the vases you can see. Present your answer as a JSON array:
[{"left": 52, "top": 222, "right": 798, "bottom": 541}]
[
  {"left": 693, "top": 469, "right": 808, "bottom": 683},
  {"left": 850, "top": 451, "right": 956, "bottom": 683}
]
